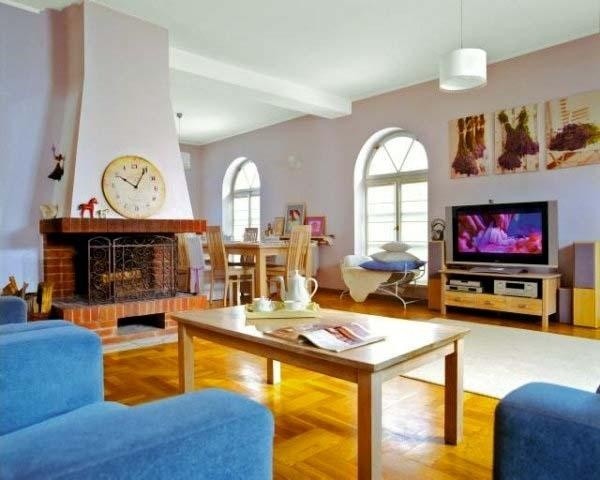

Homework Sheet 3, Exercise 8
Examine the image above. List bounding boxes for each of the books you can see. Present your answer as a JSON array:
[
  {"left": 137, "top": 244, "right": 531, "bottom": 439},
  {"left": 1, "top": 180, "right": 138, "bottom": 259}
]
[{"left": 265, "top": 315, "right": 388, "bottom": 355}]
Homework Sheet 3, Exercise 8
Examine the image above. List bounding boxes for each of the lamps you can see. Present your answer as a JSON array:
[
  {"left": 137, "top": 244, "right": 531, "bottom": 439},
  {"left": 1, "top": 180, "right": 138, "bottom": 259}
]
[
  {"left": 439, "top": 1, "right": 488, "bottom": 92},
  {"left": 177, "top": 114, "right": 191, "bottom": 171}
]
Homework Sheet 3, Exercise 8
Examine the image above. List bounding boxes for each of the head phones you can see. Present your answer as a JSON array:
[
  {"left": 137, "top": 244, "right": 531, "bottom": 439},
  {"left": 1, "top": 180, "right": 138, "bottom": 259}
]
[{"left": 430, "top": 218, "right": 446, "bottom": 240}]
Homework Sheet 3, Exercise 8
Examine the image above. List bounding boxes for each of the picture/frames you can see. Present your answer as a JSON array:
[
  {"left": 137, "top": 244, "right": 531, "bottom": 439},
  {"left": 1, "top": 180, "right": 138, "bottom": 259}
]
[
  {"left": 273, "top": 217, "right": 284, "bottom": 235},
  {"left": 545, "top": 89, "right": 600, "bottom": 170},
  {"left": 448, "top": 113, "right": 490, "bottom": 180},
  {"left": 495, "top": 102, "right": 539, "bottom": 175},
  {"left": 284, "top": 202, "right": 305, "bottom": 235},
  {"left": 304, "top": 215, "right": 328, "bottom": 236}
]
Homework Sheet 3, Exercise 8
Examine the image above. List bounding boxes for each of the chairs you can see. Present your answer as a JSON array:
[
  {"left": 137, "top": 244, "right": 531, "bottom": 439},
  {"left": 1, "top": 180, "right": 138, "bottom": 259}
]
[
  {"left": 205, "top": 226, "right": 255, "bottom": 307},
  {"left": 266, "top": 224, "right": 311, "bottom": 301}
]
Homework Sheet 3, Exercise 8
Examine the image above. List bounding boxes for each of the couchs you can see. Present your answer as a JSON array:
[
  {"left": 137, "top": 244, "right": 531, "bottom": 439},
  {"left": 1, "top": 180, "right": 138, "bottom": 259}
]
[
  {"left": 0, "top": 295, "right": 76, "bottom": 334},
  {"left": 493, "top": 382, "right": 600, "bottom": 480},
  {"left": 0, "top": 326, "right": 275, "bottom": 479}
]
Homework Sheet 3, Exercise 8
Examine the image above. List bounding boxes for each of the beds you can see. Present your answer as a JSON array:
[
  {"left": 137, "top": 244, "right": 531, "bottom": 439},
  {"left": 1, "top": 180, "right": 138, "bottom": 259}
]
[{"left": 339, "top": 243, "right": 426, "bottom": 311}]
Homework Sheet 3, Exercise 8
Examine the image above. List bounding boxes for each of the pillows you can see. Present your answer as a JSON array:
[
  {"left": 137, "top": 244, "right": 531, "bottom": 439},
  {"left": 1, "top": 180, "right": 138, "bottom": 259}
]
[
  {"left": 360, "top": 261, "right": 427, "bottom": 271},
  {"left": 370, "top": 252, "right": 419, "bottom": 263},
  {"left": 382, "top": 243, "right": 411, "bottom": 252}
]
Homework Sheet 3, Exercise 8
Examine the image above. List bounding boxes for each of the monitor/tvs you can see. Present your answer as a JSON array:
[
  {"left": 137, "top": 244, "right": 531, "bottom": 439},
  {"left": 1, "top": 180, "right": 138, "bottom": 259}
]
[{"left": 445, "top": 198, "right": 558, "bottom": 274}]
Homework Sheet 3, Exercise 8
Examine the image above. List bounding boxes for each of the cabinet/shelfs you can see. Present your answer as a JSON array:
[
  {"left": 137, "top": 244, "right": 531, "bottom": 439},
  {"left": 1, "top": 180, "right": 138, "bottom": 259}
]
[{"left": 439, "top": 268, "right": 564, "bottom": 332}]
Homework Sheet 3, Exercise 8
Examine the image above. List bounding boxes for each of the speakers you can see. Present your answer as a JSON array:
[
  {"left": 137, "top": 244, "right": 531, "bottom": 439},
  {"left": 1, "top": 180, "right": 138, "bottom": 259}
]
[
  {"left": 559, "top": 287, "right": 572, "bottom": 324},
  {"left": 572, "top": 241, "right": 596, "bottom": 290},
  {"left": 428, "top": 241, "right": 445, "bottom": 279}
]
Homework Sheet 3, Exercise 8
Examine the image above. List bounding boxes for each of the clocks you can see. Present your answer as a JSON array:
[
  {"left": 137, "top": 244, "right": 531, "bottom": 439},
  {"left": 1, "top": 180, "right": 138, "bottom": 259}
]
[{"left": 101, "top": 155, "right": 167, "bottom": 218}]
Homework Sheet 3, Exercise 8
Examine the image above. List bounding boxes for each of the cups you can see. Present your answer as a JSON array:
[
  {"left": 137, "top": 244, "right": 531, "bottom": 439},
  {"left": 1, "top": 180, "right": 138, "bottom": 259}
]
[
  {"left": 254, "top": 298, "right": 272, "bottom": 312},
  {"left": 284, "top": 300, "right": 301, "bottom": 312}
]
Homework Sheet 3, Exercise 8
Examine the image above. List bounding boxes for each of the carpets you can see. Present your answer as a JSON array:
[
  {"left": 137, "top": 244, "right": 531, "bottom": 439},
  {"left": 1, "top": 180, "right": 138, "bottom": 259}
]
[{"left": 399, "top": 317, "right": 600, "bottom": 400}]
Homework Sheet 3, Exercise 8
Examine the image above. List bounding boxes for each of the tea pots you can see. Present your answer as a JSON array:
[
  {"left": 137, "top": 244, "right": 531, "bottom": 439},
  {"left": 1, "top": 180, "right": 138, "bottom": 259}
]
[{"left": 276, "top": 270, "right": 318, "bottom": 308}]
